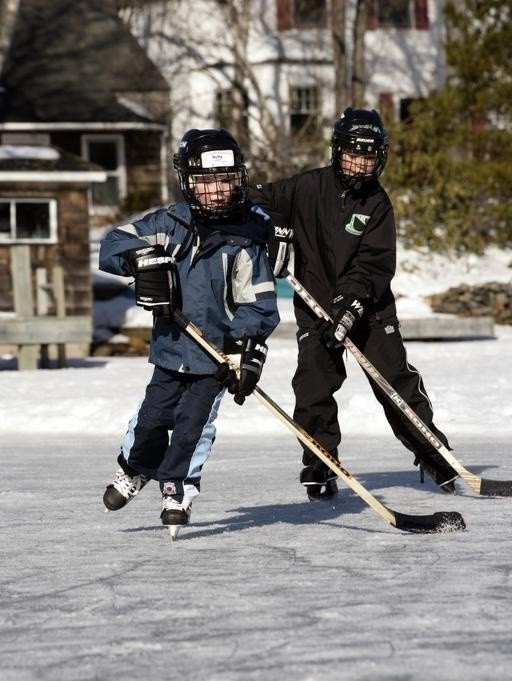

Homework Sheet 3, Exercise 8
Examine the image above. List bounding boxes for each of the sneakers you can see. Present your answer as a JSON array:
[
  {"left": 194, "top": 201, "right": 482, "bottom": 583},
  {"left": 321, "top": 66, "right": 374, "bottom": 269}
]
[
  {"left": 425, "top": 455, "right": 459, "bottom": 486},
  {"left": 103, "top": 467, "right": 151, "bottom": 510},
  {"left": 300, "top": 448, "right": 338, "bottom": 484},
  {"left": 162, "top": 495, "right": 192, "bottom": 524}
]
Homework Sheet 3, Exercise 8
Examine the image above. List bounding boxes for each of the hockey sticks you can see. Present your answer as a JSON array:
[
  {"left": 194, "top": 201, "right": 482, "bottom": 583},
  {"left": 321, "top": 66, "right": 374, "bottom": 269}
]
[
  {"left": 173, "top": 308, "right": 466, "bottom": 533},
  {"left": 282, "top": 269, "right": 512, "bottom": 497}
]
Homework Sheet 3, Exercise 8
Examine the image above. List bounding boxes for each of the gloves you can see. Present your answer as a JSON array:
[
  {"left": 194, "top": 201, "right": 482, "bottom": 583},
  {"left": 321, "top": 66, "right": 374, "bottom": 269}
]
[
  {"left": 216, "top": 332, "right": 268, "bottom": 405},
  {"left": 315, "top": 294, "right": 364, "bottom": 348},
  {"left": 264, "top": 223, "right": 294, "bottom": 277},
  {"left": 125, "top": 243, "right": 181, "bottom": 319}
]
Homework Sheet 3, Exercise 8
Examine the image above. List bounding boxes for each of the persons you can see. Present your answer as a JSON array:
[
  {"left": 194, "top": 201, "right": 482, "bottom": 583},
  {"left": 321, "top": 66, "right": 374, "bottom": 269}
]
[
  {"left": 247, "top": 106, "right": 459, "bottom": 487},
  {"left": 99, "top": 131, "right": 281, "bottom": 528}
]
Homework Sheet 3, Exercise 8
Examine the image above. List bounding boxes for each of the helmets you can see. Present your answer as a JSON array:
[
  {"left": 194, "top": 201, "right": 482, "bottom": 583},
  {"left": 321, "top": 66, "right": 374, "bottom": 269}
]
[
  {"left": 173, "top": 129, "right": 246, "bottom": 221},
  {"left": 331, "top": 106, "right": 389, "bottom": 191}
]
